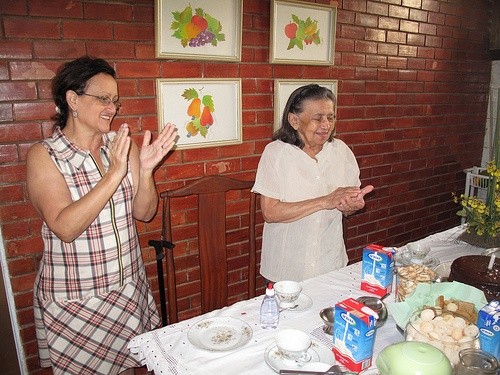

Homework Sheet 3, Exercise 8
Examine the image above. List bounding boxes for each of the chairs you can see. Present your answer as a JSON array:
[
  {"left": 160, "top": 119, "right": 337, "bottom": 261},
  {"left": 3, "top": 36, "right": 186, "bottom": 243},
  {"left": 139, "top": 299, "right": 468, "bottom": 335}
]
[{"left": 161, "top": 174, "right": 256, "bottom": 324}]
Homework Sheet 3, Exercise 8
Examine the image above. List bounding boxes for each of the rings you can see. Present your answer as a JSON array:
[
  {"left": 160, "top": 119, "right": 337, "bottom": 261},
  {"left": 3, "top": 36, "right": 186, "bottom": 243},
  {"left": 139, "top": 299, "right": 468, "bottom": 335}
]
[{"left": 161, "top": 145, "right": 164, "bottom": 149}]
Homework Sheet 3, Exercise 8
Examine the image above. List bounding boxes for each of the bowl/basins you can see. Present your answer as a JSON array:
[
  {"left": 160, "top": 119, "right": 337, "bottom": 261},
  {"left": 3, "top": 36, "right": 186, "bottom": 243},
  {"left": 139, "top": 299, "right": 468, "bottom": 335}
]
[
  {"left": 320, "top": 307, "right": 335, "bottom": 336},
  {"left": 356, "top": 297, "right": 388, "bottom": 329}
]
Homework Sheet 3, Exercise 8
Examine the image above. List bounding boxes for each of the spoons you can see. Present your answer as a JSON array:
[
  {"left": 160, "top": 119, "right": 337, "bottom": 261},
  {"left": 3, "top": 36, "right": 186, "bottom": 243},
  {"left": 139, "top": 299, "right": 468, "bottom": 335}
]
[{"left": 279, "top": 305, "right": 299, "bottom": 313}]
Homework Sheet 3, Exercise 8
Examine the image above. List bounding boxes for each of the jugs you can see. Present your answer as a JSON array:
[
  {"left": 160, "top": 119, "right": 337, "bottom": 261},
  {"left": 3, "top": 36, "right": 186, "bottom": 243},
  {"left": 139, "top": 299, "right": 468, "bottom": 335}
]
[{"left": 455, "top": 348, "right": 500, "bottom": 375}]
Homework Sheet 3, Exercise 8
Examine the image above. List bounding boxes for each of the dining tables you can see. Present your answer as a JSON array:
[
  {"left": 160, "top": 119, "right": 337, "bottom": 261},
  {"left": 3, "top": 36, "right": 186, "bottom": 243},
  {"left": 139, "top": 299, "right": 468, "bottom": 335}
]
[{"left": 131, "top": 224, "right": 500, "bottom": 375}]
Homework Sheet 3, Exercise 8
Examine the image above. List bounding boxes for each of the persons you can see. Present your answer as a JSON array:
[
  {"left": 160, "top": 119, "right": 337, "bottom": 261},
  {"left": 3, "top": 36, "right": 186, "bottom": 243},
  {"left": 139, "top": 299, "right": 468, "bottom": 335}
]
[
  {"left": 26, "top": 56, "right": 179, "bottom": 375},
  {"left": 251, "top": 84, "right": 374, "bottom": 287}
]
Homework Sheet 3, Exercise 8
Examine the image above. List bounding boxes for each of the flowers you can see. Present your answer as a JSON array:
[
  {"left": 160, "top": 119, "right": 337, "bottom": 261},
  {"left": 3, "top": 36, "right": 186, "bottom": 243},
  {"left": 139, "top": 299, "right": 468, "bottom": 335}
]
[{"left": 451, "top": 163, "right": 500, "bottom": 236}]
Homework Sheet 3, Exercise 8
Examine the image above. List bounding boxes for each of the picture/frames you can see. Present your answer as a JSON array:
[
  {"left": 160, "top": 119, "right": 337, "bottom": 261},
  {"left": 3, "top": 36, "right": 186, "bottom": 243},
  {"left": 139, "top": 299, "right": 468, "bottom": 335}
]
[
  {"left": 274, "top": 78, "right": 337, "bottom": 137},
  {"left": 156, "top": 78, "right": 243, "bottom": 151},
  {"left": 154, "top": 0, "right": 243, "bottom": 63},
  {"left": 269, "top": 0, "right": 336, "bottom": 66}
]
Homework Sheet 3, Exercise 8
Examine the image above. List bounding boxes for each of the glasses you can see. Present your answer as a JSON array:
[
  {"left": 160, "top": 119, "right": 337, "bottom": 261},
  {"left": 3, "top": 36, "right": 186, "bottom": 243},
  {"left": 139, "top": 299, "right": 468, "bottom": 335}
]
[{"left": 75, "top": 92, "right": 121, "bottom": 109}]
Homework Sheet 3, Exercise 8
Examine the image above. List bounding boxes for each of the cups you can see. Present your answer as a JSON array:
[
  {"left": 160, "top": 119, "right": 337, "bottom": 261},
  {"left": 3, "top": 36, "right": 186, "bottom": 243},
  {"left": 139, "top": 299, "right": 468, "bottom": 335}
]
[
  {"left": 275, "top": 329, "right": 312, "bottom": 362},
  {"left": 407, "top": 242, "right": 431, "bottom": 263},
  {"left": 273, "top": 280, "right": 303, "bottom": 306}
]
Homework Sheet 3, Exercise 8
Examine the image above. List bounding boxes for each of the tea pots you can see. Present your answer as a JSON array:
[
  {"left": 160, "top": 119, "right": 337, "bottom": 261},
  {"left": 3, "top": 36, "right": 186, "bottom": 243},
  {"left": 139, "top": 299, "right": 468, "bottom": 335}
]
[{"left": 376, "top": 341, "right": 453, "bottom": 375}]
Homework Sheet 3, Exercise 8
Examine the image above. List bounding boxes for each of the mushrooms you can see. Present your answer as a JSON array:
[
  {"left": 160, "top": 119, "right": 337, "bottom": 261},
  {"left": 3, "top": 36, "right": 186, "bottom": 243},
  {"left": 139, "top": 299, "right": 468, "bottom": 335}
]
[{"left": 406, "top": 308, "right": 479, "bottom": 364}]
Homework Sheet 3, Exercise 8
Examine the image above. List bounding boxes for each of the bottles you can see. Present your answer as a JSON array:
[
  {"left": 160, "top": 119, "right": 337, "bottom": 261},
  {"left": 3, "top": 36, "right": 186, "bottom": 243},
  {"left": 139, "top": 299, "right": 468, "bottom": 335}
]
[
  {"left": 404, "top": 309, "right": 481, "bottom": 375},
  {"left": 259, "top": 283, "right": 280, "bottom": 329}
]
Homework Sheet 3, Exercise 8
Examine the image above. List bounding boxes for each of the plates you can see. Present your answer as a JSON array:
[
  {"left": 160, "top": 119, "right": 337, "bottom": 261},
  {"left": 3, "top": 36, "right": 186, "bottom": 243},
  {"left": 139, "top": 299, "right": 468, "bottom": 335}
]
[
  {"left": 188, "top": 317, "right": 253, "bottom": 352},
  {"left": 265, "top": 340, "right": 335, "bottom": 375},
  {"left": 395, "top": 250, "right": 440, "bottom": 269},
  {"left": 275, "top": 294, "right": 313, "bottom": 313}
]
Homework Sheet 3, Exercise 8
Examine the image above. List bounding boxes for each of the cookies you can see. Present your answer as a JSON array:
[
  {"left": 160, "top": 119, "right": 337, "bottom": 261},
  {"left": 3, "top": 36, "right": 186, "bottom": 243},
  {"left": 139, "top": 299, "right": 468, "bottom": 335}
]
[
  {"left": 423, "top": 296, "right": 478, "bottom": 324},
  {"left": 398, "top": 265, "right": 438, "bottom": 301}
]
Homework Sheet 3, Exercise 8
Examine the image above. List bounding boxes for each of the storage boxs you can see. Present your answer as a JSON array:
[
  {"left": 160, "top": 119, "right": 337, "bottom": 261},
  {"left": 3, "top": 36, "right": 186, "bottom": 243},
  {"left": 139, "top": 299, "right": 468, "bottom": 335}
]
[
  {"left": 478, "top": 300, "right": 500, "bottom": 362},
  {"left": 361, "top": 243, "right": 397, "bottom": 295},
  {"left": 332, "top": 298, "right": 378, "bottom": 371}
]
